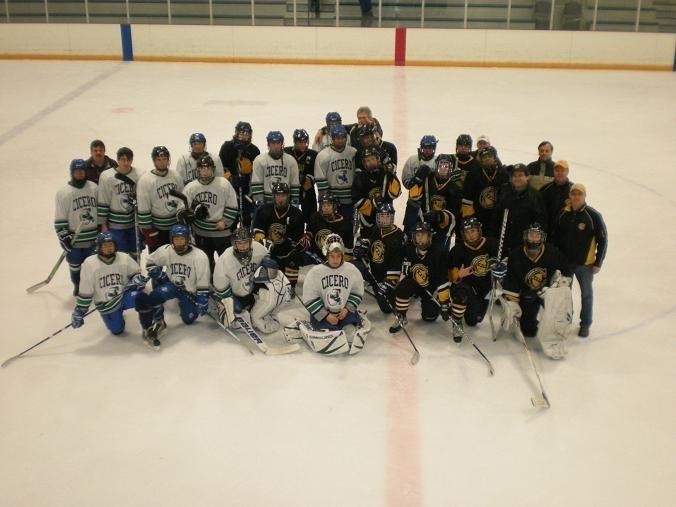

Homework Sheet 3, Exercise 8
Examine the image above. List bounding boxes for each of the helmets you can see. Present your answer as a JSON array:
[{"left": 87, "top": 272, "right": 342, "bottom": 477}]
[
  {"left": 233, "top": 121, "right": 255, "bottom": 144},
  {"left": 192, "top": 156, "right": 347, "bottom": 217},
  {"left": 324, "top": 110, "right": 348, "bottom": 138},
  {"left": 293, "top": 127, "right": 309, "bottom": 141},
  {"left": 354, "top": 126, "right": 384, "bottom": 174},
  {"left": 94, "top": 223, "right": 252, "bottom": 254},
  {"left": 373, "top": 199, "right": 549, "bottom": 251},
  {"left": 69, "top": 157, "right": 87, "bottom": 170},
  {"left": 188, "top": 131, "right": 207, "bottom": 144},
  {"left": 266, "top": 130, "right": 285, "bottom": 144},
  {"left": 320, "top": 232, "right": 348, "bottom": 257},
  {"left": 417, "top": 132, "right": 499, "bottom": 183},
  {"left": 150, "top": 144, "right": 171, "bottom": 158}
]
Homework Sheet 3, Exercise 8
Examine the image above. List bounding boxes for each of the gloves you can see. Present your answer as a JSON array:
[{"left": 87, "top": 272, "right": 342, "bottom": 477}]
[
  {"left": 140, "top": 225, "right": 161, "bottom": 245},
  {"left": 423, "top": 208, "right": 446, "bottom": 225},
  {"left": 147, "top": 265, "right": 172, "bottom": 285},
  {"left": 414, "top": 162, "right": 432, "bottom": 185},
  {"left": 127, "top": 271, "right": 146, "bottom": 287},
  {"left": 56, "top": 226, "right": 75, "bottom": 254},
  {"left": 68, "top": 304, "right": 89, "bottom": 329},
  {"left": 490, "top": 260, "right": 508, "bottom": 279},
  {"left": 194, "top": 294, "right": 211, "bottom": 316}
]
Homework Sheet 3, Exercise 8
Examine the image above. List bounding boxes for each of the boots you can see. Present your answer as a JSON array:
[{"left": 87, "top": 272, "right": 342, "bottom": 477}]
[
  {"left": 387, "top": 309, "right": 410, "bottom": 335},
  {"left": 140, "top": 327, "right": 164, "bottom": 352},
  {"left": 151, "top": 317, "right": 168, "bottom": 330},
  {"left": 451, "top": 316, "right": 465, "bottom": 343}
]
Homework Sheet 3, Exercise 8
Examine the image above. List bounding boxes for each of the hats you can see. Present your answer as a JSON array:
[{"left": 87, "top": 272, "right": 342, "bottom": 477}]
[
  {"left": 474, "top": 134, "right": 493, "bottom": 145},
  {"left": 569, "top": 181, "right": 588, "bottom": 194},
  {"left": 510, "top": 161, "right": 532, "bottom": 175},
  {"left": 551, "top": 159, "right": 571, "bottom": 169}
]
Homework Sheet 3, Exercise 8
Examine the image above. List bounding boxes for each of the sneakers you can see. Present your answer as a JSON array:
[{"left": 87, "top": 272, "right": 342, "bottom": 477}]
[{"left": 577, "top": 324, "right": 590, "bottom": 338}]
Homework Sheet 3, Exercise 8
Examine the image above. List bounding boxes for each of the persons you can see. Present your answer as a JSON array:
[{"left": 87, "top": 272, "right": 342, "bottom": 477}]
[{"left": 53, "top": 105, "right": 608, "bottom": 355}]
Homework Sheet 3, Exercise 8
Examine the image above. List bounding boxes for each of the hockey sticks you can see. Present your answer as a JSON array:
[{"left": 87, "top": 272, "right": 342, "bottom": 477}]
[
  {"left": 499, "top": 295, "right": 552, "bottom": 408},
  {"left": 424, "top": 286, "right": 494, "bottom": 375},
  {"left": 162, "top": 278, "right": 256, "bottom": 357},
  {"left": 0, "top": 278, "right": 151, "bottom": 368},
  {"left": 26, "top": 222, "right": 83, "bottom": 294},
  {"left": 361, "top": 257, "right": 420, "bottom": 364},
  {"left": 233, "top": 312, "right": 300, "bottom": 355},
  {"left": 474, "top": 207, "right": 509, "bottom": 328}
]
